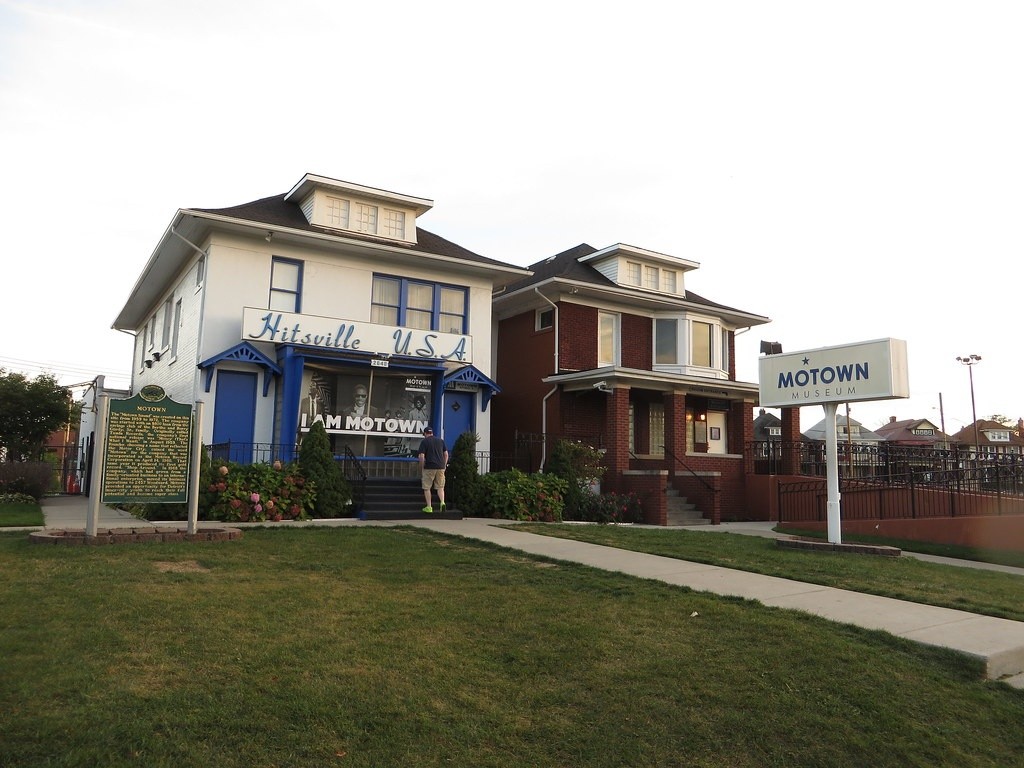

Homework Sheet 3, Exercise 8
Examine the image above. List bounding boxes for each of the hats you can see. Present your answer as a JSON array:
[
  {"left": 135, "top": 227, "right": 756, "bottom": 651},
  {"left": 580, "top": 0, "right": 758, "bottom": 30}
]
[{"left": 423, "top": 428, "right": 432, "bottom": 434}]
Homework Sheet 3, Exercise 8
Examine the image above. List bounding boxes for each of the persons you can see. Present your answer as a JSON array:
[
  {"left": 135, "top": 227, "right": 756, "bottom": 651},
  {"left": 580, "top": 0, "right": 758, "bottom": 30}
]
[
  {"left": 417, "top": 427, "right": 449, "bottom": 512},
  {"left": 384, "top": 410, "right": 391, "bottom": 420},
  {"left": 297, "top": 380, "right": 326, "bottom": 435},
  {"left": 342, "top": 384, "right": 378, "bottom": 428},
  {"left": 408, "top": 396, "right": 428, "bottom": 456}
]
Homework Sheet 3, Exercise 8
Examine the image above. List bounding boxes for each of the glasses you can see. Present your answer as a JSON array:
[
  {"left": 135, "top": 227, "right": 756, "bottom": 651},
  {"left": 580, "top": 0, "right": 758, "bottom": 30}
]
[{"left": 355, "top": 394, "right": 367, "bottom": 398}]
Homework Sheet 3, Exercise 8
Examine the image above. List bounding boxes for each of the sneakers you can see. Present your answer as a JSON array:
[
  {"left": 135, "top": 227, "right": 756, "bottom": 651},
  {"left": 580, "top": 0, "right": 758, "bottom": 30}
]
[
  {"left": 422, "top": 507, "right": 432, "bottom": 512},
  {"left": 440, "top": 502, "right": 446, "bottom": 513}
]
